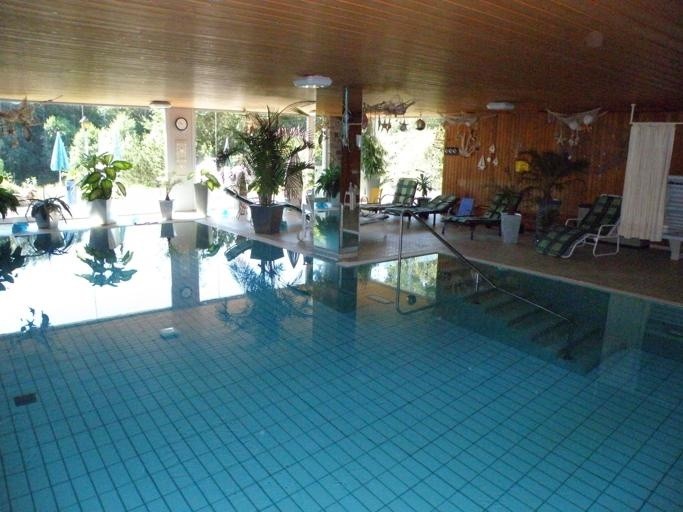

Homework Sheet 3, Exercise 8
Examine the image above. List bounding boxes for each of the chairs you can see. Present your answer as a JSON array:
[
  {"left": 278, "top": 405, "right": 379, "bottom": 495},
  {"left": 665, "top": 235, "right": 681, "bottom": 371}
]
[{"left": 358, "top": 176, "right": 624, "bottom": 262}]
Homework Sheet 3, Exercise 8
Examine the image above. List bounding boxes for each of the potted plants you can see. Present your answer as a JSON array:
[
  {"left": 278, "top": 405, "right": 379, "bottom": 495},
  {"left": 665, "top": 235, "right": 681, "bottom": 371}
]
[
  {"left": 75, "top": 152, "right": 131, "bottom": 225},
  {"left": 159, "top": 223, "right": 186, "bottom": 258},
  {"left": 25, "top": 233, "right": 75, "bottom": 258},
  {"left": 213, "top": 241, "right": 321, "bottom": 353},
  {"left": 211, "top": 99, "right": 317, "bottom": 234},
  {"left": 22, "top": 195, "right": 72, "bottom": 229},
  {"left": 516, "top": 148, "right": 591, "bottom": 242},
  {"left": 0, "top": 235, "right": 27, "bottom": 292},
  {"left": 187, "top": 167, "right": 220, "bottom": 219},
  {"left": 74, "top": 226, "right": 138, "bottom": 287},
  {"left": 157, "top": 176, "right": 183, "bottom": 221},
  {"left": 317, "top": 161, "right": 342, "bottom": 204},
  {"left": 189, "top": 223, "right": 220, "bottom": 260}
]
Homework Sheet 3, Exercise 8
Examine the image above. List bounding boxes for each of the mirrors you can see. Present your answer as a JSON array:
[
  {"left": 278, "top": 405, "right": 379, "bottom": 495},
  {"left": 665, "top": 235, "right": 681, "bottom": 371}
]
[{"left": 316, "top": 85, "right": 360, "bottom": 254}]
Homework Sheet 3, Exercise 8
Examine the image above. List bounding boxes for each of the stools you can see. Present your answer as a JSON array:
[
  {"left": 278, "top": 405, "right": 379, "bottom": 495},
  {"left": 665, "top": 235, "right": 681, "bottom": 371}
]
[{"left": 342, "top": 191, "right": 358, "bottom": 211}]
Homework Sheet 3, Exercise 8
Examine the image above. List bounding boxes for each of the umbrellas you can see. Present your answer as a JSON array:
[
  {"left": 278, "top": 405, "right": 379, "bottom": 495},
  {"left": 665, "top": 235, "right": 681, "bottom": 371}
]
[{"left": 49, "top": 131, "right": 69, "bottom": 183}]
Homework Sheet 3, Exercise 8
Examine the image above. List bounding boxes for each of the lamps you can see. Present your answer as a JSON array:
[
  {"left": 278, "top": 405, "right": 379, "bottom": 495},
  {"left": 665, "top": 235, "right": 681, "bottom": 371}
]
[
  {"left": 414, "top": 109, "right": 424, "bottom": 130},
  {"left": 149, "top": 101, "right": 172, "bottom": 109},
  {"left": 487, "top": 102, "right": 513, "bottom": 111},
  {"left": 292, "top": 76, "right": 333, "bottom": 90},
  {"left": 157, "top": 327, "right": 175, "bottom": 340}
]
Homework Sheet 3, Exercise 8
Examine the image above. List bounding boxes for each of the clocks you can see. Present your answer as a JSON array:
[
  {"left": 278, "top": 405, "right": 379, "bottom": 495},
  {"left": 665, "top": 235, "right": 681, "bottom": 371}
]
[
  {"left": 179, "top": 286, "right": 192, "bottom": 299},
  {"left": 175, "top": 116, "right": 187, "bottom": 131}
]
